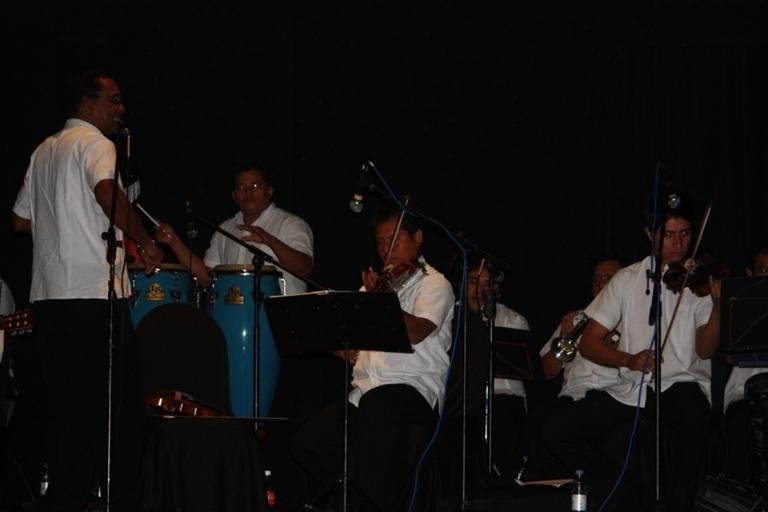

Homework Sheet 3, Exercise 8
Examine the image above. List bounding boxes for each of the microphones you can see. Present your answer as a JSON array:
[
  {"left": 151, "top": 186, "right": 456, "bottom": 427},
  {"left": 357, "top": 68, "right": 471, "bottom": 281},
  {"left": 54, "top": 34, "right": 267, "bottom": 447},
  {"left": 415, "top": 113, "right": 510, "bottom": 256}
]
[
  {"left": 662, "top": 170, "right": 682, "bottom": 210},
  {"left": 183, "top": 197, "right": 200, "bottom": 239},
  {"left": 348, "top": 161, "right": 369, "bottom": 214}
]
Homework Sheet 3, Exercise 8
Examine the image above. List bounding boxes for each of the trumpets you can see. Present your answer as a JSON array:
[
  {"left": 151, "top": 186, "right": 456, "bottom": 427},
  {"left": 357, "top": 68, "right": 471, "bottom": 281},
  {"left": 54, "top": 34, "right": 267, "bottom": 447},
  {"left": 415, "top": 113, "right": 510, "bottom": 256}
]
[{"left": 552, "top": 318, "right": 588, "bottom": 362}]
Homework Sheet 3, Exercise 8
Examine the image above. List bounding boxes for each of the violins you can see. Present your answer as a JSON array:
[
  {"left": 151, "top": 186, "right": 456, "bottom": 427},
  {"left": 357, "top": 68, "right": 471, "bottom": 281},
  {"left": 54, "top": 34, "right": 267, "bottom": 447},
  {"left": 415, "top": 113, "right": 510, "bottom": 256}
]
[
  {"left": 662, "top": 253, "right": 764, "bottom": 297},
  {"left": 372, "top": 260, "right": 415, "bottom": 292},
  {"left": 145, "top": 391, "right": 266, "bottom": 439}
]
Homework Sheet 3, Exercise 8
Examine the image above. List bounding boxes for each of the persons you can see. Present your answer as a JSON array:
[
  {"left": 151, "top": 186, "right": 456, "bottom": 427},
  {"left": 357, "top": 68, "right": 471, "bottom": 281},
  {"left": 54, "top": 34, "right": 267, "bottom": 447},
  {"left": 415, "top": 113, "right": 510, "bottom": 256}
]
[
  {"left": 155, "top": 163, "right": 314, "bottom": 295},
  {"left": 539, "top": 208, "right": 768, "bottom": 511},
  {"left": 336, "top": 213, "right": 530, "bottom": 451},
  {"left": 0, "top": 77, "right": 161, "bottom": 512}
]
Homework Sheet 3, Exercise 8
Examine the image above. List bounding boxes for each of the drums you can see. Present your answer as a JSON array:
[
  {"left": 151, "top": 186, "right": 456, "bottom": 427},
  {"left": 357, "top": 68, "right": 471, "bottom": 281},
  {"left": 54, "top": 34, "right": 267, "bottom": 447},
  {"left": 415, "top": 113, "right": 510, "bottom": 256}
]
[
  {"left": 206, "top": 264, "right": 283, "bottom": 417},
  {"left": 127, "top": 262, "right": 199, "bottom": 330}
]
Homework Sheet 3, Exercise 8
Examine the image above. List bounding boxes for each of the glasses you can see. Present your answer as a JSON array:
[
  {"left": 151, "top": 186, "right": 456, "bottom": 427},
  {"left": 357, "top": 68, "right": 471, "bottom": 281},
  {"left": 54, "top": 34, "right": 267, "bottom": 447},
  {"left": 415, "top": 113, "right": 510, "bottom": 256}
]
[{"left": 234, "top": 181, "right": 263, "bottom": 192}]
